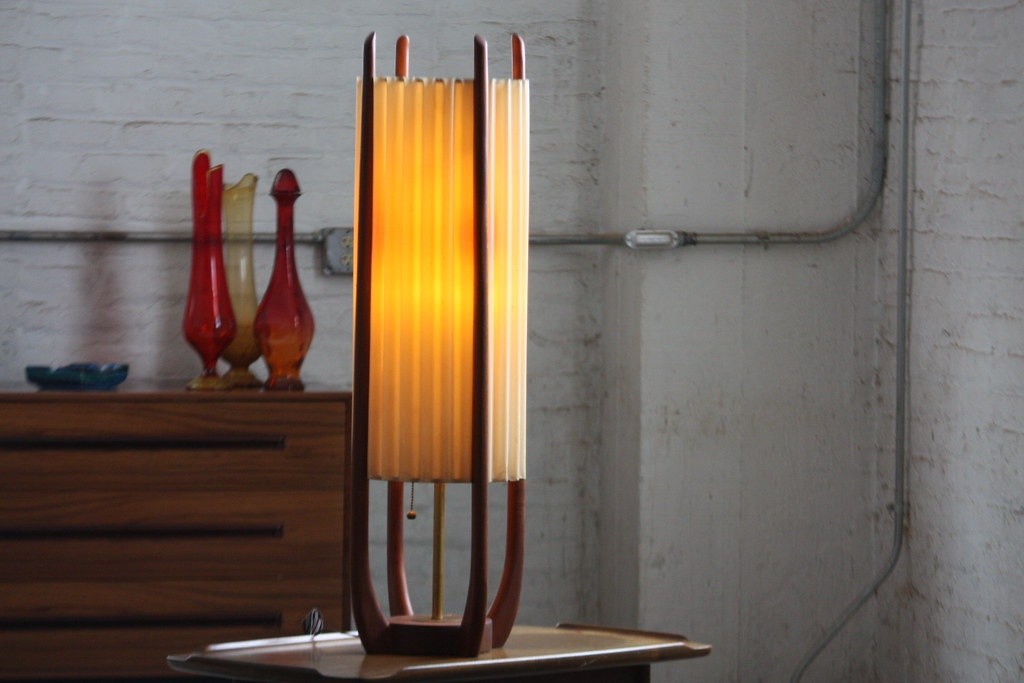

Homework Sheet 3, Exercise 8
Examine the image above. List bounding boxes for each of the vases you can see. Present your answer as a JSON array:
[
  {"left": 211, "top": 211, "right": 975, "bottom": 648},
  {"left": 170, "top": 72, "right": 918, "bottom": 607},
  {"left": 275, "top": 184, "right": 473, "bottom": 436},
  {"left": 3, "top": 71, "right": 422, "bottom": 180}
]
[
  {"left": 221, "top": 172, "right": 263, "bottom": 388},
  {"left": 254, "top": 168, "right": 314, "bottom": 391},
  {"left": 181, "top": 150, "right": 238, "bottom": 390}
]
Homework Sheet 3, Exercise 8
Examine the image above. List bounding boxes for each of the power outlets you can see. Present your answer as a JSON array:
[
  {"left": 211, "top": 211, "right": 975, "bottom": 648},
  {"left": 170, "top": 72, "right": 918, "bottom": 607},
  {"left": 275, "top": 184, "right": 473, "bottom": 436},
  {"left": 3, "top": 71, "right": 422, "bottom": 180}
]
[{"left": 320, "top": 227, "right": 354, "bottom": 276}]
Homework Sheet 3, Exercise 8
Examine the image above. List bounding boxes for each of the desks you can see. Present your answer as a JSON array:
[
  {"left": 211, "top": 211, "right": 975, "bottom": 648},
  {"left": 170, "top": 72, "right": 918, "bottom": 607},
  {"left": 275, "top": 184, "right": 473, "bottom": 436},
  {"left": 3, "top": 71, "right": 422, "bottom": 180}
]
[{"left": 167, "top": 616, "right": 713, "bottom": 683}]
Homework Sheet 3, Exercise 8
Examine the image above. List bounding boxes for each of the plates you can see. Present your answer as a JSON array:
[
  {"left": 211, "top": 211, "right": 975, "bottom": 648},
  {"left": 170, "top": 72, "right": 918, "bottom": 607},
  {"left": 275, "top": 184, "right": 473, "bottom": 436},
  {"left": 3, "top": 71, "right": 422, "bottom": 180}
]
[{"left": 24, "top": 363, "right": 129, "bottom": 389}]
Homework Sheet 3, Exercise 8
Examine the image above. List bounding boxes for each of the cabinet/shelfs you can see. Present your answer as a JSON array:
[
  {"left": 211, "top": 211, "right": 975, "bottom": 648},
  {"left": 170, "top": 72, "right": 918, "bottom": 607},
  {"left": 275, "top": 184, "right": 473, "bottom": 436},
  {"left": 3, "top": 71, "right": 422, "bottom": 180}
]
[{"left": 0, "top": 382, "right": 353, "bottom": 683}]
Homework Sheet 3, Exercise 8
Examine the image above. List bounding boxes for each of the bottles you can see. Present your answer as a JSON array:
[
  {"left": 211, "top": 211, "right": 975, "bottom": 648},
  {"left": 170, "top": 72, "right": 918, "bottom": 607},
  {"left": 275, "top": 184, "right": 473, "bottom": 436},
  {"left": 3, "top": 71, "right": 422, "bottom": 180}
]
[
  {"left": 253, "top": 168, "right": 313, "bottom": 391},
  {"left": 182, "top": 149, "right": 236, "bottom": 391},
  {"left": 221, "top": 174, "right": 261, "bottom": 387}
]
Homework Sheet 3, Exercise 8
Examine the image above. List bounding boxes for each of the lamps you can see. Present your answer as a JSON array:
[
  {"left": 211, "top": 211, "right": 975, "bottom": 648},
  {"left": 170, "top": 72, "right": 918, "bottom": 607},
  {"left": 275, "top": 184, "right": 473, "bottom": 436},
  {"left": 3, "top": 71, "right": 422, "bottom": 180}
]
[{"left": 342, "top": 33, "right": 531, "bottom": 655}]
